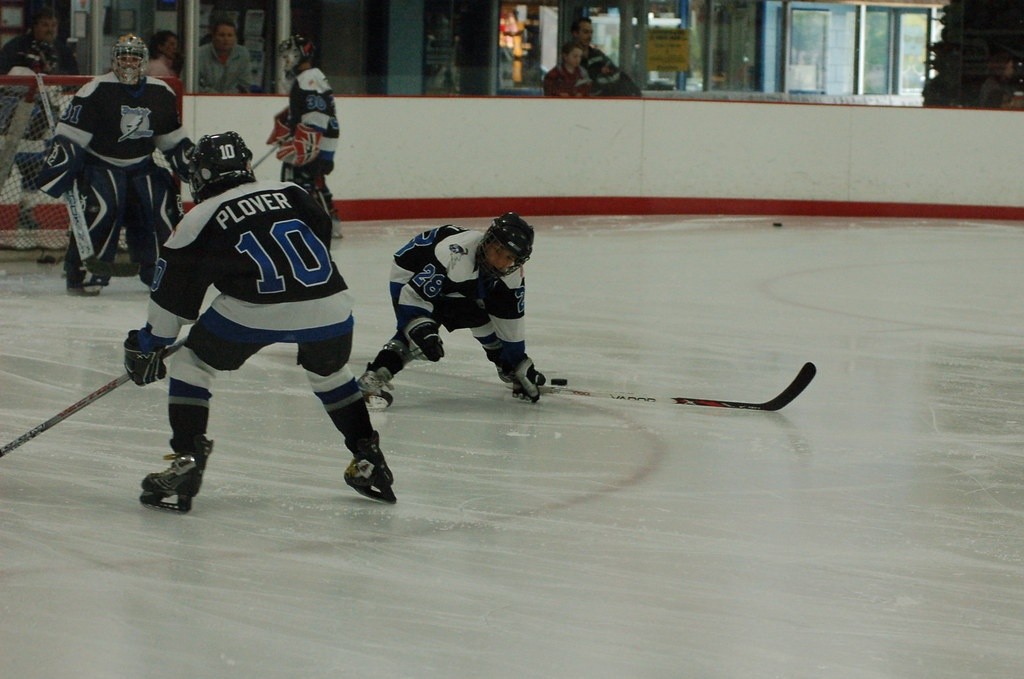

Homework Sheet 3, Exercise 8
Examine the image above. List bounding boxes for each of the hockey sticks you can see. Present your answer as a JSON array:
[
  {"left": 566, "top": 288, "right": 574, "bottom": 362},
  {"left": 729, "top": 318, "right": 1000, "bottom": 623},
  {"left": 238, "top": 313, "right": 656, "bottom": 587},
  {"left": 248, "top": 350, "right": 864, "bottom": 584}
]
[
  {"left": 0, "top": 339, "right": 193, "bottom": 461},
  {"left": 514, "top": 361, "right": 817, "bottom": 419},
  {"left": 37, "top": 79, "right": 144, "bottom": 277}
]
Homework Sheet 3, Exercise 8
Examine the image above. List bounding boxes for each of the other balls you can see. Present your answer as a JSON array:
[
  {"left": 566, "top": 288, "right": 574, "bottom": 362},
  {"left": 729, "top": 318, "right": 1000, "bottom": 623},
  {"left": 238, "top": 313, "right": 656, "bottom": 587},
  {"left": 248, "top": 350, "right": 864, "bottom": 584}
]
[
  {"left": 773, "top": 221, "right": 782, "bottom": 227},
  {"left": 551, "top": 379, "right": 567, "bottom": 386}
]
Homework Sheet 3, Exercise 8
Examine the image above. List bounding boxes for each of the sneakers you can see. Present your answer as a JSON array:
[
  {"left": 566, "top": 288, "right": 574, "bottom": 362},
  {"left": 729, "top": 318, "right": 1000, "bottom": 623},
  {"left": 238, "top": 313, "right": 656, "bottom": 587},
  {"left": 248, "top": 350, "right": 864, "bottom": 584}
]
[
  {"left": 67, "top": 286, "right": 100, "bottom": 297},
  {"left": 496, "top": 365, "right": 546, "bottom": 401},
  {"left": 344, "top": 430, "right": 397, "bottom": 504},
  {"left": 140, "top": 439, "right": 214, "bottom": 515},
  {"left": 357, "top": 361, "right": 404, "bottom": 409}
]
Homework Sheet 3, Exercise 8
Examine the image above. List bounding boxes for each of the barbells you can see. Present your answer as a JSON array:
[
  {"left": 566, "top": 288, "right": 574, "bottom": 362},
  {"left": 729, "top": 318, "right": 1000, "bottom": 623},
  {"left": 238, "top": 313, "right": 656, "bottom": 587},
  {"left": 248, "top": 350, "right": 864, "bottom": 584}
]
[{"left": 246, "top": 144, "right": 284, "bottom": 170}]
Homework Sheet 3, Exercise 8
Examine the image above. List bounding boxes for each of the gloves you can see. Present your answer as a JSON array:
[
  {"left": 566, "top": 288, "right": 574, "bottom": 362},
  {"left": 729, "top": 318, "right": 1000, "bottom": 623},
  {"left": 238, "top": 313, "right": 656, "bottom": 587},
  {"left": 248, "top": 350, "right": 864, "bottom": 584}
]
[
  {"left": 512, "top": 357, "right": 539, "bottom": 404},
  {"left": 123, "top": 329, "right": 166, "bottom": 386},
  {"left": 404, "top": 318, "right": 444, "bottom": 362}
]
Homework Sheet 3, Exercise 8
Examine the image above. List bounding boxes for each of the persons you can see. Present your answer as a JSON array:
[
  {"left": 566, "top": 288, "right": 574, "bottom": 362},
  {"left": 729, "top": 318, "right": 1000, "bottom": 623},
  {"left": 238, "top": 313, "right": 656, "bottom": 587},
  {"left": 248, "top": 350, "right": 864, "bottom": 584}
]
[
  {"left": 924, "top": 29, "right": 1004, "bottom": 108},
  {"left": 0, "top": 5, "right": 75, "bottom": 75},
  {"left": 196, "top": 19, "right": 253, "bottom": 94},
  {"left": 122, "top": 131, "right": 393, "bottom": 497},
  {"left": 443, "top": 31, "right": 485, "bottom": 95},
  {"left": 544, "top": 42, "right": 591, "bottom": 95},
  {"left": 148, "top": 30, "right": 180, "bottom": 77},
  {"left": 356, "top": 210, "right": 547, "bottom": 408},
  {"left": 37, "top": 33, "right": 197, "bottom": 287},
  {"left": 570, "top": 17, "right": 620, "bottom": 96},
  {"left": 269, "top": 35, "right": 344, "bottom": 239}
]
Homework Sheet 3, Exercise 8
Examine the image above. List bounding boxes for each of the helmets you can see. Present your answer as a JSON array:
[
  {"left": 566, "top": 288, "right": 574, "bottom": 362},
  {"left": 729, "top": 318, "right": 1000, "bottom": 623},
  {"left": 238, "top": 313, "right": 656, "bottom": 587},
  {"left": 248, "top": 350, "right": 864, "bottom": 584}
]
[
  {"left": 277, "top": 35, "right": 315, "bottom": 78},
  {"left": 188, "top": 130, "right": 257, "bottom": 204},
  {"left": 477, "top": 211, "right": 534, "bottom": 277},
  {"left": 113, "top": 34, "right": 149, "bottom": 85}
]
